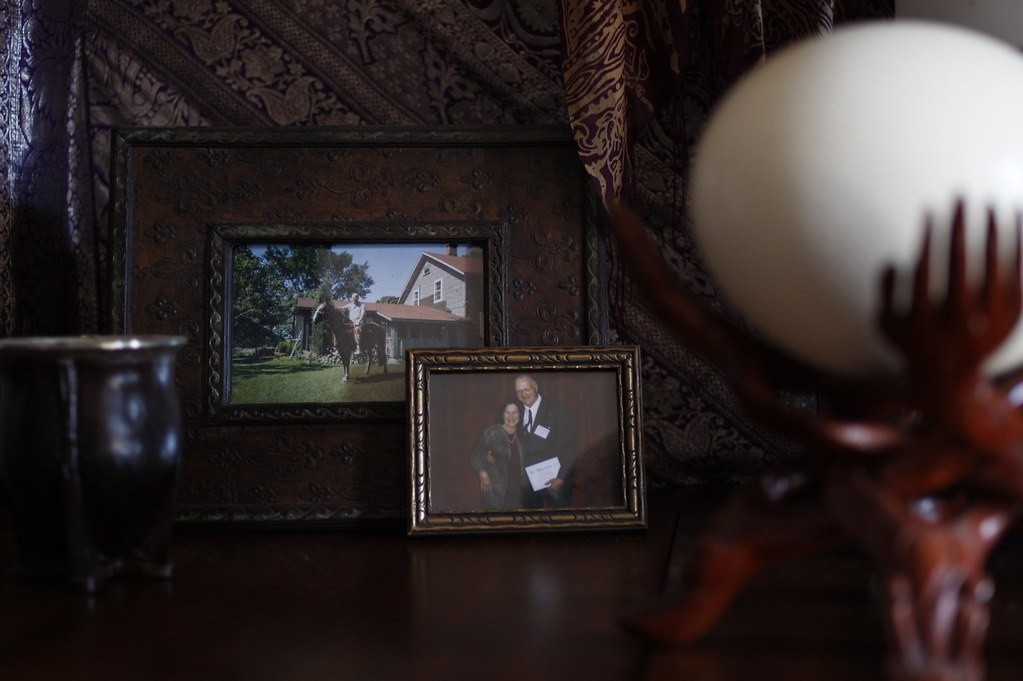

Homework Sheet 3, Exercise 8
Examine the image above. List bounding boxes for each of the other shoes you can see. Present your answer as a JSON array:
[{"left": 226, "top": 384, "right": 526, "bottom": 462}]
[{"left": 354, "top": 349, "right": 360, "bottom": 355}]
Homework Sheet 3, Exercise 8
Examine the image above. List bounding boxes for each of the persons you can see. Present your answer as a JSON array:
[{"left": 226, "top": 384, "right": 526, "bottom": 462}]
[
  {"left": 467, "top": 372, "right": 580, "bottom": 515},
  {"left": 336, "top": 292, "right": 367, "bottom": 355}
]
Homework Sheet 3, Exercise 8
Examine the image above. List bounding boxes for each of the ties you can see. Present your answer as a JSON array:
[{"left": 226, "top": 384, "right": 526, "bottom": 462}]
[{"left": 524, "top": 409, "right": 532, "bottom": 433}]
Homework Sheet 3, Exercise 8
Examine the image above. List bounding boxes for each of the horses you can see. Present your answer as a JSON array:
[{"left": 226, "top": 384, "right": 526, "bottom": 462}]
[{"left": 312, "top": 301, "right": 388, "bottom": 383}]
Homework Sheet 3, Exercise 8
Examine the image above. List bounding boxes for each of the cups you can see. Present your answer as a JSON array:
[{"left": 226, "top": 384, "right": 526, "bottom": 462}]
[{"left": 0, "top": 335, "right": 190, "bottom": 592}]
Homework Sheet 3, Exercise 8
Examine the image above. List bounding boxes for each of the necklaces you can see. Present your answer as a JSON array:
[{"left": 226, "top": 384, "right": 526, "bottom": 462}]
[{"left": 503, "top": 429, "right": 516, "bottom": 444}]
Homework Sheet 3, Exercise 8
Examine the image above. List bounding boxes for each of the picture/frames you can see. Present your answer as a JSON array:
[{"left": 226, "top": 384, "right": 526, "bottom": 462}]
[
  {"left": 103, "top": 125, "right": 613, "bottom": 540},
  {"left": 405, "top": 344, "right": 649, "bottom": 540}
]
